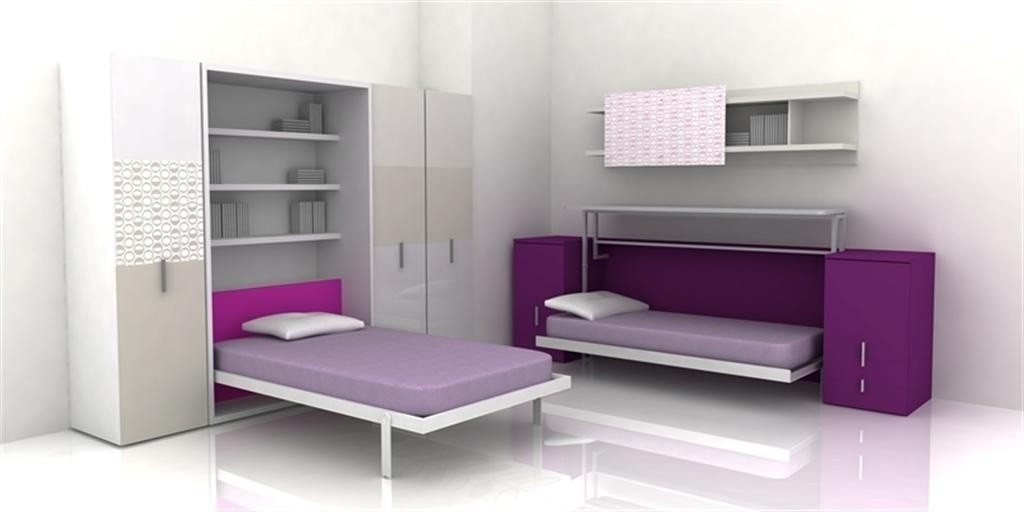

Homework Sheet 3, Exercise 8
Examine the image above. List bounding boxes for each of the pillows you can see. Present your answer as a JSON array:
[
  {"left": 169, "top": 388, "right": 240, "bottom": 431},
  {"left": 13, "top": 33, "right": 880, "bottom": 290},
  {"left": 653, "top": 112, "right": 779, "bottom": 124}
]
[
  {"left": 540, "top": 287, "right": 650, "bottom": 322},
  {"left": 239, "top": 307, "right": 366, "bottom": 341}
]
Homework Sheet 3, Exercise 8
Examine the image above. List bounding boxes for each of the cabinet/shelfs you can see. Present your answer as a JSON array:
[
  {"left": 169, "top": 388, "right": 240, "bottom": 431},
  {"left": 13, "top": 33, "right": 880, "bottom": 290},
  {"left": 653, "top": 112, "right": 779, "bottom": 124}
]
[
  {"left": 823, "top": 246, "right": 935, "bottom": 417},
  {"left": 61, "top": 56, "right": 208, "bottom": 447},
  {"left": 586, "top": 78, "right": 866, "bottom": 170},
  {"left": 314, "top": 84, "right": 429, "bottom": 335},
  {"left": 421, "top": 90, "right": 472, "bottom": 340},
  {"left": 511, "top": 233, "right": 583, "bottom": 363},
  {"left": 201, "top": 68, "right": 370, "bottom": 253}
]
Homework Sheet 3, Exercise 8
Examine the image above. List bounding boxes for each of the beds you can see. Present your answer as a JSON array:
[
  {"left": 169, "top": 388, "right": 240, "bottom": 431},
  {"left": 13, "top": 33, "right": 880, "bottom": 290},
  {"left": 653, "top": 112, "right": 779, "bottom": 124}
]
[
  {"left": 536, "top": 306, "right": 821, "bottom": 385},
  {"left": 212, "top": 278, "right": 570, "bottom": 475}
]
